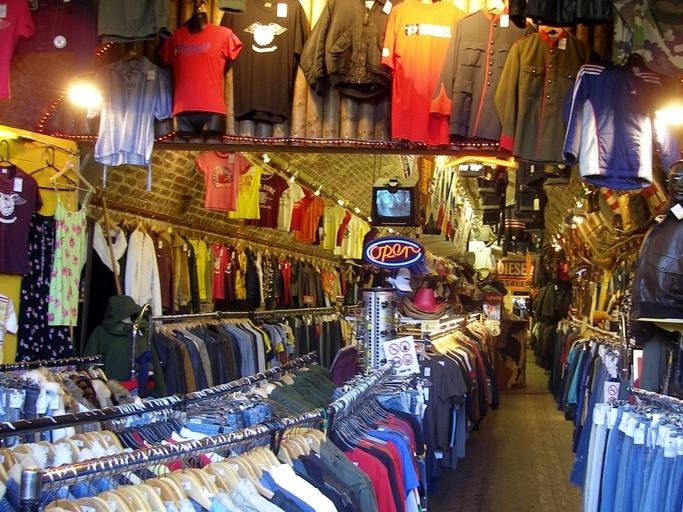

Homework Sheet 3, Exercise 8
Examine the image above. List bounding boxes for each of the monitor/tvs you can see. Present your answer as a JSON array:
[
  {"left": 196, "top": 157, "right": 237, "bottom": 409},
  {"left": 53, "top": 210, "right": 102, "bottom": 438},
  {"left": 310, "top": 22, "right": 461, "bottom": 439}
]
[{"left": 372, "top": 186, "right": 420, "bottom": 227}]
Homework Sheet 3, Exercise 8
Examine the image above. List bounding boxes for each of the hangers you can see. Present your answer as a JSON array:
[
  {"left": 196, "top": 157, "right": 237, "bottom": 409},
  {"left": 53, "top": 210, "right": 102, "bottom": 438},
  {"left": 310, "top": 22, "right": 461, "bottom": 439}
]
[
  {"left": 414, "top": 323, "right": 484, "bottom": 363},
  {"left": 119, "top": 41, "right": 145, "bottom": 63},
  {"left": 481, "top": 2, "right": 568, "bottom": 37}
]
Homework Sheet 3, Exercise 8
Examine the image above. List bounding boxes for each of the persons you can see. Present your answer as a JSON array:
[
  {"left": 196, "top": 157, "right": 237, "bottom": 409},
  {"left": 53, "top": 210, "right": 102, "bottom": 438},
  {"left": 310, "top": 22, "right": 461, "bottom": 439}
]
[{"left": 157, "top": 9, "right": 240, "bottom": 136}]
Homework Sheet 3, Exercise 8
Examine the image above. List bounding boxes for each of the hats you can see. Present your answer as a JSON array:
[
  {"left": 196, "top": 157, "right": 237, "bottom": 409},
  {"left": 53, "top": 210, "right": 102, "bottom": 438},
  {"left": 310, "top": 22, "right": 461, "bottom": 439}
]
[
  {"left": 385, "top": 269, "right": 413, "bottom": 293},
  {"left": 417, "top": 251, "right": 474, "bottom": 284},
  {"left": 402, "top": 287, "right": 447, "bottom": 319}
]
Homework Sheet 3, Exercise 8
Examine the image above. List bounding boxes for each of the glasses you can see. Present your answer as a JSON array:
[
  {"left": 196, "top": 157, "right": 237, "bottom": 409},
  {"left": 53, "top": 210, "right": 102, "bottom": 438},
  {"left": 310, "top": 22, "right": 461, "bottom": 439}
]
[
  {"left": 380, "top": 329, "right": 396, "bottom": 337},
  {"left": 381, "top": 301, "right": 397, "bottom": 308}
]
